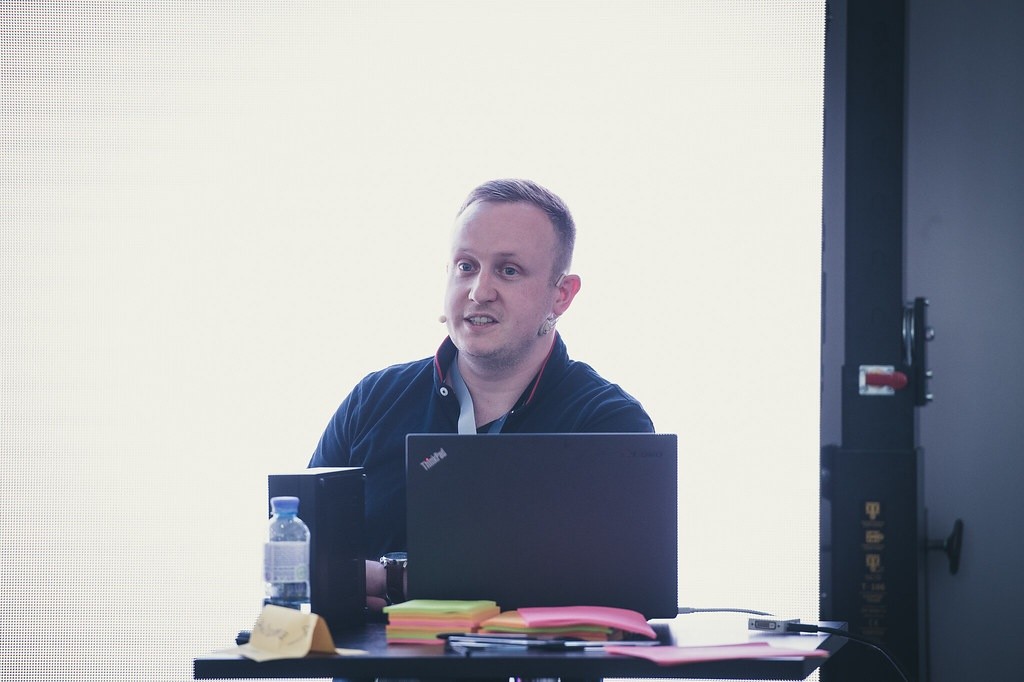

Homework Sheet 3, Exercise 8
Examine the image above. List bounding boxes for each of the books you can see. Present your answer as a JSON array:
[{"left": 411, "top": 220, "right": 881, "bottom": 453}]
[{"left": 382, "top": 595, "right": 657, "bottom": 648}]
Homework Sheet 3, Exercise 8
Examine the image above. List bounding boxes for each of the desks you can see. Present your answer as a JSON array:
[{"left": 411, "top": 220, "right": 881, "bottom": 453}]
[{"left": 194, "top": 622, "right": 851, "bottom": 682}]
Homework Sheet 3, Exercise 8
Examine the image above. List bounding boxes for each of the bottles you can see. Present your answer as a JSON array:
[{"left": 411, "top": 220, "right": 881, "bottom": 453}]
[{"left": 261, "top": 496, "right": 311, "bottom": 614}]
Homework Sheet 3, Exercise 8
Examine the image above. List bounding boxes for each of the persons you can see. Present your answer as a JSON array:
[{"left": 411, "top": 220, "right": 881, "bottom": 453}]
[{"left": 306, "top": 178, "right": 655, "bottom": 624}]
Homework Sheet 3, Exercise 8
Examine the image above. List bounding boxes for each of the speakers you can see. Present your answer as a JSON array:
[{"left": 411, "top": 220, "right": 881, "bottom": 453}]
[{"left": 266, "top": 467, "right": 367, "bottom": 633}]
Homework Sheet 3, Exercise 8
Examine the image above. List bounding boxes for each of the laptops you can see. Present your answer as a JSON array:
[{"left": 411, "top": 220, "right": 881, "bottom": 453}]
[{"left": 403, "top": 433, "right": 679, "bottom": 621}]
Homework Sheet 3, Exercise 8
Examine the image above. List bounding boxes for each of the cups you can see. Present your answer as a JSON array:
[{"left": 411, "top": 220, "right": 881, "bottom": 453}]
[{"left": 381, "top": 551, "right": 408, "bottom": 606}]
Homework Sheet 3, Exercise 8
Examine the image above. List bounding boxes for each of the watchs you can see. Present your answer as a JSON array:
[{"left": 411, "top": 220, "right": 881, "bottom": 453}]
[{"left": 379, "top": 551, "right": 408, "bottom": 605}]
